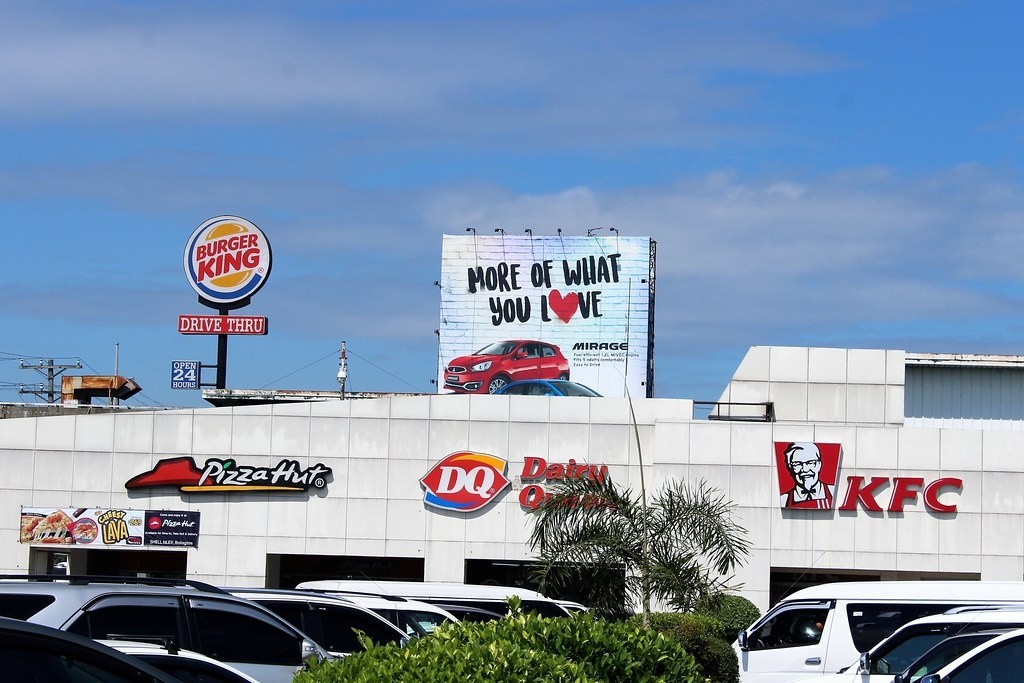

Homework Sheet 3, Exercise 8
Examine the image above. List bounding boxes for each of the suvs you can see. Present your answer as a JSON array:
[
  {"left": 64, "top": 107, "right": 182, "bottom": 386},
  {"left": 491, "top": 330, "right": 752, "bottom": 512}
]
[
  {"left": 795, "top": 605, "right": 1024, "bottom": 683},
  {"left": 0, "top": 572, "right": 338, "bottom": 683},
  {"left": 291, "top": 586, "right": 469, "bottom": 650}
]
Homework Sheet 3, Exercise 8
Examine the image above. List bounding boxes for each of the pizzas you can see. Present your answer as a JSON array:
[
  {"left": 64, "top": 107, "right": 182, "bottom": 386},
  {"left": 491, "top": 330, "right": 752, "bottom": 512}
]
[{"left": 19, "top": 510, "right": 96, "bottom": 543}]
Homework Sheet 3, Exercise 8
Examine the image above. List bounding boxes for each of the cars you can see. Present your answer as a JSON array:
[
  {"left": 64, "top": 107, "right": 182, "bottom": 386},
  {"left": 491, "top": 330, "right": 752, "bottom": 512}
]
[
  {"left": 443, "top": 339, "right": 570, "bottom": 400},
  {"left": 1, "top": 613, "right": 178, "bottom": 683},
  {"left": 554, "top": 599, "right": 600, "bottom": 627},
  {"left": 498, "top": 378, "right": 602, "bottom": 400},
  {"left": 428, "top": 603, "right": 506, "bottom": 634},
  {"left": 219, "top": 586, "right": 415, "bottom": 663},
  {"left": 913, "top": 628, "right": 1024, "bottom": 683}
]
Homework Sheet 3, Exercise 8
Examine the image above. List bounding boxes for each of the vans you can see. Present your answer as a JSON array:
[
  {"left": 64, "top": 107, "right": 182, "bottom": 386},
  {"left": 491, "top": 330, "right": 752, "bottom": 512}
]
[
  {"left": 295, "top": 580, "right": 575, "bottom": 632},
  {"left": 732, "top": 581, "right": 1024, "bottom": 683}
]
